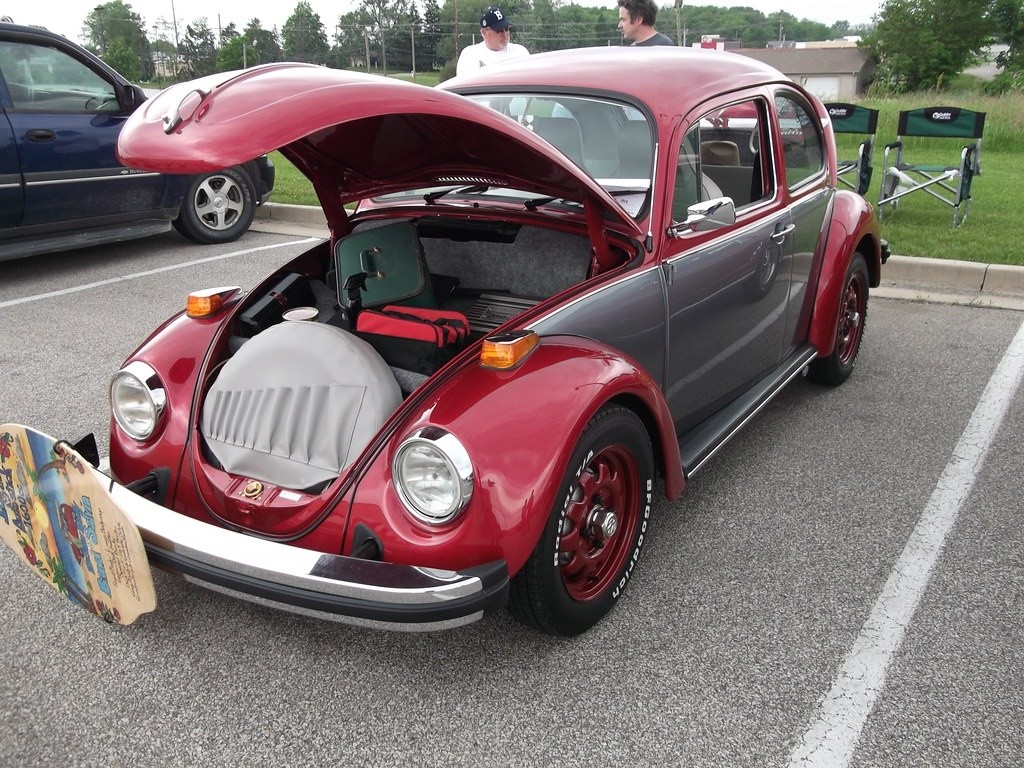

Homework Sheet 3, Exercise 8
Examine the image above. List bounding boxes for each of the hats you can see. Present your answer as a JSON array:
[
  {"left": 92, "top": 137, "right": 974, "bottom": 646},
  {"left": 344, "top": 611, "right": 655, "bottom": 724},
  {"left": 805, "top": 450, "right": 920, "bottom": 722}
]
[{"left": 480, "top": 7, "right": 513, "bottom": 30}]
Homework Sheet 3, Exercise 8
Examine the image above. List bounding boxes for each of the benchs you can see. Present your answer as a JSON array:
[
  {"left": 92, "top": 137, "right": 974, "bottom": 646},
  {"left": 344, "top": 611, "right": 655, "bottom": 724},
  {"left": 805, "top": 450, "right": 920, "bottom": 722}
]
[{"left": 697, "top": 139, "right": 813, "bottom": 204}]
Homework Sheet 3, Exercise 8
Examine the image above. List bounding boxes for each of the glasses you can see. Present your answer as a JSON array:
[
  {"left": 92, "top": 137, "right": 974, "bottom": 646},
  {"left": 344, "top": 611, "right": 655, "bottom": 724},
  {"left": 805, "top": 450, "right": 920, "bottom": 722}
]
[{"left": 484, "top": 25, "right": 509, "bottom": 34}]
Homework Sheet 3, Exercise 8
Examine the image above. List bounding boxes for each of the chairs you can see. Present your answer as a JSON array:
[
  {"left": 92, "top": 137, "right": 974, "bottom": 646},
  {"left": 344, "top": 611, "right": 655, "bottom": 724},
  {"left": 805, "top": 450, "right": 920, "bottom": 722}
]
[
  {"left": 618, "top": 120, "right": 724, "bottom": 222},
  {"left": 823, "top": 102, "right": 879, "bottom": 196},
  {"left": 533, "top": 115, "right": 583, "bottom": 156},
  {"left": 879, "top": 106, "right": 987, "bottom": 230}
]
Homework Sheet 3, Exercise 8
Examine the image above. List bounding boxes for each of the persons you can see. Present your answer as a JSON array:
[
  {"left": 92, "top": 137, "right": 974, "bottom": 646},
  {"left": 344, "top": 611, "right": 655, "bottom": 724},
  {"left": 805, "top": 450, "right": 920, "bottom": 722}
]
[
  {"left": 617, "top": 0, "right": 675, "bottom": 119},
  {"left": 457, "top": 7, "right": 530, "bottom": 116}
]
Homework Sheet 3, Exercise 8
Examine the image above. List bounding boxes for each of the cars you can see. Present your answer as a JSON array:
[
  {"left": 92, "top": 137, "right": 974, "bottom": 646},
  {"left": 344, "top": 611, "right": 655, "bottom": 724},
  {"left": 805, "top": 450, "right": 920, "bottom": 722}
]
[
  {"left": 0, "top": 18, "right": 277, "bottom": 260},
  {"left": 83, "top": 44, "right": 893, "bottom": 638}
]
